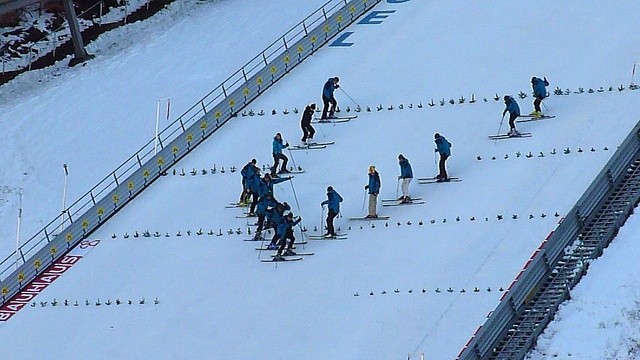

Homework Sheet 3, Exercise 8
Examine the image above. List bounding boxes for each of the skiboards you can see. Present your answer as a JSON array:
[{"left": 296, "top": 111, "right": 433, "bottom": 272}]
[
  {"left": 488, "top": 132, "right": 533, "bottom": 139},
  {"left": 247, "top": 221, "right": 266, "bottom": 226},
  {"left": 226, "top": 203, "right": 253, "bottom": 208},
  {"left": 268, "top": 170, "right": 304, "bottom": 179},
  {"left": 287, "top": 141, "right": 335, "bottom": 150},
  {"left": 254, "top": 242, "right": 307, "bottom": 250},
  {"left": 236, "top": 212, "right": 257, "bottom": 219},
  {"left": 244, "top": 227, "right": 281, "bottom": 241},
  {"left": 349, "top": 216, "right": 391, "bottom": 221},
  {"left": 311, "top": 116, "right": 358, "bottom": 123},
  {"left": 517, "top": 114, "right": 556, "bottom": 123},
  {"left": 379, "top": 197, "right": 424, "bottom": 206},
  {"left": 417, "top": 177, "right": 462, "bottom": 184},
  {"left": 261, "top": 252, "right": 314, "bottom": 262},
  {"left": 308, "top": 234, "right": 348, "bottom": 241}
]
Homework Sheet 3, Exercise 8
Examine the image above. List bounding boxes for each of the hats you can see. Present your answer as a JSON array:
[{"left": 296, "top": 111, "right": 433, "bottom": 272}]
[
  {"left": 311, "top": 103, "right": 316, "bottom": 109},
  {"left": 369, "top": 166, "right": 375, "bottom": 174}
]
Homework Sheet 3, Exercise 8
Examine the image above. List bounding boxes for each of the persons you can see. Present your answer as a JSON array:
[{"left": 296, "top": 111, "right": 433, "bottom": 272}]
[
  {"left": 298, "top": 103, "right": 318, "bottom": 148},
  {"left": 321, "top": 185, "right": 343, "bottom": 239},
  {"left": 320, "top": 77, "right": 339, "bottom": 119},
  {"left": 270, "top": 132, "right": 289, "bottom": 177},
  {"left": 397, "top": 153, "right": 414, "bottom": 204},
  {"left": 240, "top": 159, "right": 302, "bottom": 260},
  {"left": 530, "top": 77, "right": 550, "bottom": 120},
  {"left": 503, "top": 95, "right": 521, "bottom": 137},
  {"left": 434, "top": 132, "right": 451, "bottom": 182},
  {"left": 365, "top": 165, "right": 381, "bottom": 219}
]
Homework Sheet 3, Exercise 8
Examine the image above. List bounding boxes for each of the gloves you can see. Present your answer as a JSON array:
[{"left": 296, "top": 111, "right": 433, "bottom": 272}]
[
  {"left": 286, "top": 143, "right": 289, "bottom": 146},
  {"left": 365, "top": 186, "right": 369, "bottom": 190}
]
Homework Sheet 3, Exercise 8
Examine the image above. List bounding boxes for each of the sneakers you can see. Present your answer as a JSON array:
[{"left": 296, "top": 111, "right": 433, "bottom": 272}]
[
  {"left": 300, "top": 141, "right": 308, "bottom": 145},
  {"left": 255, "top": 234, "right": 262, "bottom": 239},
  {"left": 285, "top": 249, "right": 296, "bottom": 255},
  {"left": 509, "top": 130, "right": 517, "bottom": 136},
  {"left": 529, "top": 109, "right": 537, "bottom": 115},
  {"left": 308, "top": 138, "right": 317, "bottom": 144},
  {"left": 324, "top": 233, "right": 333, "bottom": 238},
  {"left": 268, "top": 242, "right": 279, "bottom": 249},
  {"left": 403, "top": 197, "right": 412, "bottom": 202},
  {"left": 365, "top": 214, "right": 378, "bottom": 218},
  {"left": 533, "top": 112, "right": 541, "bottom": 118},
  {"left": 274, "top": 255, "right": 285, "bottom": 261}
]
